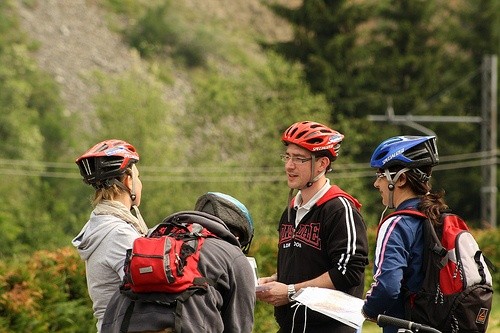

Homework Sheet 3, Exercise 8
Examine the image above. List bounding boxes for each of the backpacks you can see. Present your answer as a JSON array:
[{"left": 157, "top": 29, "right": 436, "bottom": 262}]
[
  {"left": 376, "top": 207, "right": 495, "bottom": 333},
  {"left": 119, "top": 216, "right": 221, "bottom": 306}
]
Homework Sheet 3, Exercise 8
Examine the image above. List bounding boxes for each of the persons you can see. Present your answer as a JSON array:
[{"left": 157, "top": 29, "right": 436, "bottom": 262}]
[
  {"left": 362, "top": 134, "right": 449, "bottom": 333},
  {"left": 100, "top": 192, "right": 256, "bottom": 333},
  {"left": 257, "top": 120, "right": 369, "bottom": 333},
  {"left": 73, "top": 139, "right": 149, "bottom": 333}
]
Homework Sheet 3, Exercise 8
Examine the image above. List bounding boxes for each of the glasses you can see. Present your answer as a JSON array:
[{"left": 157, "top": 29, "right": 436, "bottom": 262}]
[{"left": 279, "top": 154, "right": 324, "bottom": 165}]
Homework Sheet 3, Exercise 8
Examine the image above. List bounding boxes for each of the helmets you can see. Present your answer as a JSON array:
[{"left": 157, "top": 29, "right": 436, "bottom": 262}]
[
  {"left": 194, "top": 192, "right": 254, "bottom": 254},
  {"left": 75, "top": 139, "right": 139, "bottom": 184},
  {"left": 281, "top": 121, "right": 345, "bottom": 162},
  {"left": 370, "top": 135, "right": 440, "bottom": 169}
]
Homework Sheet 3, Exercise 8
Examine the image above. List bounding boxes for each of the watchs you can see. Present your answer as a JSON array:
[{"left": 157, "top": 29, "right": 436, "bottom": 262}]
[{"left": 287, "top": 284, "right": 297, "bottom": 302}]
